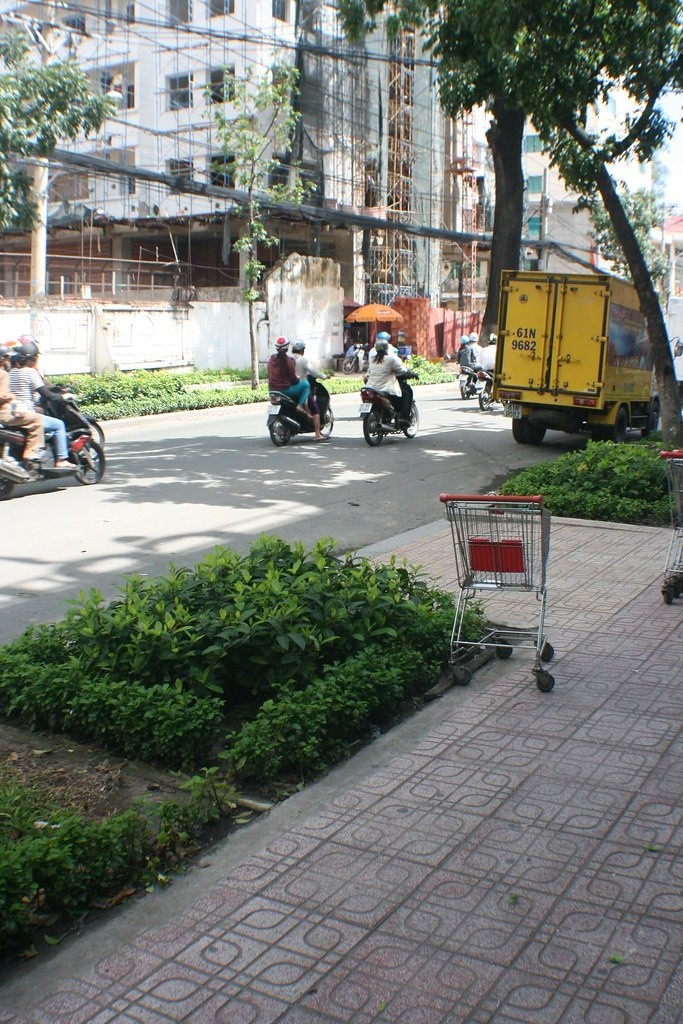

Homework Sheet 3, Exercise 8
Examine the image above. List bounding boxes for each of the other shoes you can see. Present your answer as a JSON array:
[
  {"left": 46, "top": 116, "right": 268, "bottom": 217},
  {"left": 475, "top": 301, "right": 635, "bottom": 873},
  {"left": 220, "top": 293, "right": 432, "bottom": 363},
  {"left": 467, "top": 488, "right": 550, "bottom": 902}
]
[
  {"left": 397, "top": 417, "right": 413, "bottom": 424},
  {"left": 55, "top": 460, "right": 76, "bottom": 467},
  {"left": 22, "top": 450, "right": 41, "bottom": 459}
]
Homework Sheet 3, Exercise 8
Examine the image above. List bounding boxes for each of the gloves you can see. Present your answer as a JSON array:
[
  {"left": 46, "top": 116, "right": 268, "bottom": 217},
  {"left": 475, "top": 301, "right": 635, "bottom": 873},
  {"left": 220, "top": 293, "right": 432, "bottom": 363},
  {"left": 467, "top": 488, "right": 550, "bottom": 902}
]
[{"left": 408, "top": 370, "right": 420, "bottom": 380}]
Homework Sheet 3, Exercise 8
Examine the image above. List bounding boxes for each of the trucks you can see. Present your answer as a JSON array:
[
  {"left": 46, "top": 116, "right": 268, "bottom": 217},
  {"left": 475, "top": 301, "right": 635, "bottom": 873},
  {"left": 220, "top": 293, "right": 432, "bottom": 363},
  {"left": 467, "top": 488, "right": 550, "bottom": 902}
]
[{"left": 491, "top": 268, "right": 683, "bottom": 447}]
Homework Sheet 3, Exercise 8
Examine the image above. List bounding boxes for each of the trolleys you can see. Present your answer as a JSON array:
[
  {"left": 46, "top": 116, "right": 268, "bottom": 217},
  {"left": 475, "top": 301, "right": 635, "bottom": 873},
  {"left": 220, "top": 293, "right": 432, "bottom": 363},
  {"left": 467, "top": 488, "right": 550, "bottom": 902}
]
[
  {"left": 439, "top": 493, "right": 555, "bottom": 692},
  {"left": 660, "top": 449, "right": 683, "bottom": 606}
]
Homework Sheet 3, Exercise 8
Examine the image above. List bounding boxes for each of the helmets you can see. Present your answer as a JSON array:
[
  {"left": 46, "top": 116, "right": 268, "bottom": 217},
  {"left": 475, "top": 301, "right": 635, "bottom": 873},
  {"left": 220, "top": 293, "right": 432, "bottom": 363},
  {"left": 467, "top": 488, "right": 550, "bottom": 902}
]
[
  {"left": 0, "top": 344, "right": 18, "bottom": 357},
  {"left": 19, "top": 335, "right": 39, "bottom": 346},
  {"left": 374, "top": 340, "right": 388, "bottom": 352},
  {"left": 292, "top": 340, "right": 305, "bottom": 351},
  {"left": 469, "top": 332, "right": 479, "bottom": 341},
  {"left": 18, "top": 341, "right": 41, "bottom": 357},
  {"left": 377, "top": 331, "right": 391, "bottom": 341},
  {"left": 487, "top": 333, "right": 497, "bottom": 343},
  {"left": 460, "top": 335, "right": 469, "bottom": 345},
  {"left": 274, "top": 336, "right": 290, "bottom": 350}
]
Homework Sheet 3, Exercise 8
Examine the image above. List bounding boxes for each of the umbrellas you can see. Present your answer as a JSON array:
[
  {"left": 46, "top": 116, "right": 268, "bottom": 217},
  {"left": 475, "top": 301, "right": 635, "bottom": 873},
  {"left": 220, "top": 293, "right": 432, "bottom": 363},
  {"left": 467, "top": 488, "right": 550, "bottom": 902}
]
[{"left": 346, "top": 304, "right": 403, "bottom": 338}]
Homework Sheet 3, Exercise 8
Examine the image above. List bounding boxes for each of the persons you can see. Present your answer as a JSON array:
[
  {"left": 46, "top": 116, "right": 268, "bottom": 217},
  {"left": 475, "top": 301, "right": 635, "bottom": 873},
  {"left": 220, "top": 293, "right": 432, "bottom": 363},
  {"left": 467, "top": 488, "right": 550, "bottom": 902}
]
[
  {"left": 346, "top": 331, "right": 392, "bottom": 373},
  {"left": 287, "top": 339, "right": 329, "bottom": 441},
  {"left": 457, "top": 332, "right": 496, "bottom": 400},
  {"left": 268, "top": 336, "right": 311, "bottom": 415},
  {"left": 0, "top": 336, "right": 78, "bottom": 469},
  {"left": 365, "top": 340, "right": 419, "bottom": 426}
]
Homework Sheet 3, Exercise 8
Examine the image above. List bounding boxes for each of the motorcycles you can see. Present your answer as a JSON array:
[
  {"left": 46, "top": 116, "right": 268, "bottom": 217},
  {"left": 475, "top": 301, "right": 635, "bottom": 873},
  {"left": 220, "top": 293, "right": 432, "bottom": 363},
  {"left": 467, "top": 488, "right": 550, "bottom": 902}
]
[
  {"left": 458, "top": 365, "right": 477, "bottom": 400},
  {"left": 0, "top": 382, "right": 107, "bottom": 499},
  {"left": 476, "top": 371, "right": 494, "bottom": 412},
  {"left": 266, "top": 374, "right": 335, "bottom": 447},
  {"left": 358, "top": 369, "right": 421, "bottom": 446}
]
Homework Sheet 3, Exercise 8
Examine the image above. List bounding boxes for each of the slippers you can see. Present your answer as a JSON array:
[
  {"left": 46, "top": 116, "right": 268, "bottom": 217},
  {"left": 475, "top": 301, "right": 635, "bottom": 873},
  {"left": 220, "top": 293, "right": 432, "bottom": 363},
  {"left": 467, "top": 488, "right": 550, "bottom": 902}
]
[
  {"left": 296, "top": 407, "right": 308, "bottom": 415},
  {"left": 316, "top": 436, "right": 330, "bottom": 442}
]
